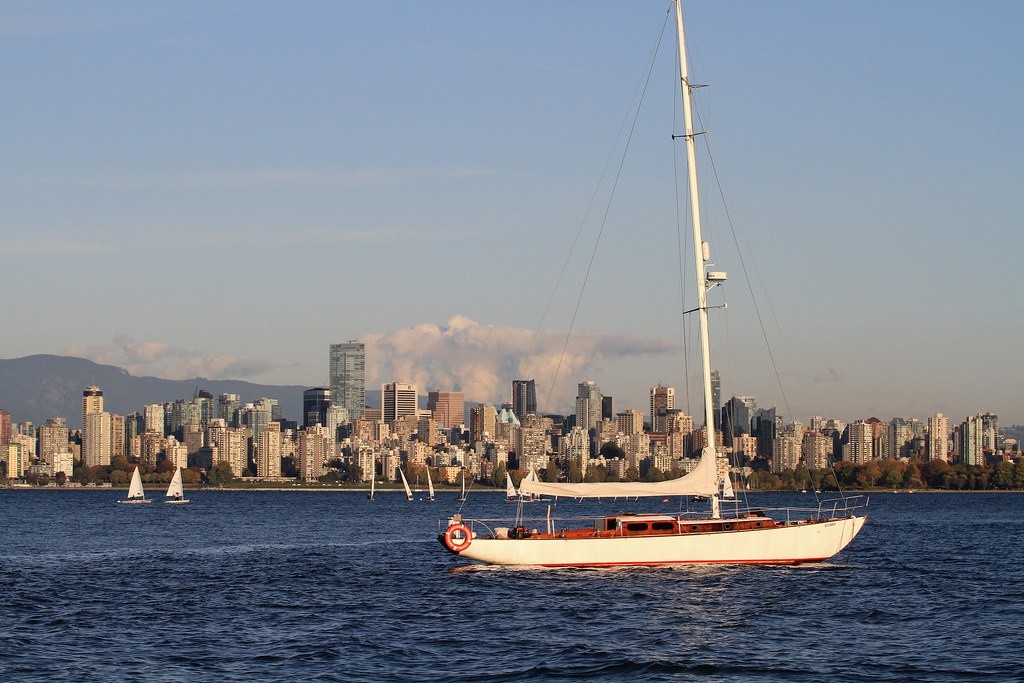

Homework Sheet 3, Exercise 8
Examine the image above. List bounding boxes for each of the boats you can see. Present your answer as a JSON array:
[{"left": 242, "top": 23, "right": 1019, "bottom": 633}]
[{"left": 816, "top": 491, "right": 821, "bottom": 494}]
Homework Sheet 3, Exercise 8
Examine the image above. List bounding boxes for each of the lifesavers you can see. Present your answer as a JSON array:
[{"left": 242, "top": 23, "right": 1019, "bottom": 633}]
[{"left": 444, "top": 523, "right": 471, "bottom": 552}]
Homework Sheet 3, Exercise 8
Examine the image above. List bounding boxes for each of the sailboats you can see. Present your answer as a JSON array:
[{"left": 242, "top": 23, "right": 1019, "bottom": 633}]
[
  {"left": 437, "top": 1, "right": 868, "bottom": 564},
  {"left": 399, "top": 467, "right": 414, "bottom": 501},
  {"left": 720, "top": 467, "right": 742, "bottom": 503},
  {"left": 503, "top": 470, "right": 551, "bottom": 502},
  {"left": 163, "top": 466, "right": 190, "bottom": 505},
  {"left": 425, "top": 465, "right": 435, "bottom": 502},
  {"left": 367, "top": 444, "right": 376, "bottom": 500},
  {"left": 457, "top": 468, "right": 467, "bottom": 502},
  {"left": 117, "top": 466, "right": 152, "bottom": 504},
  {"left": 801, "top": 479, "right": 806, "bottom": 493}
]
